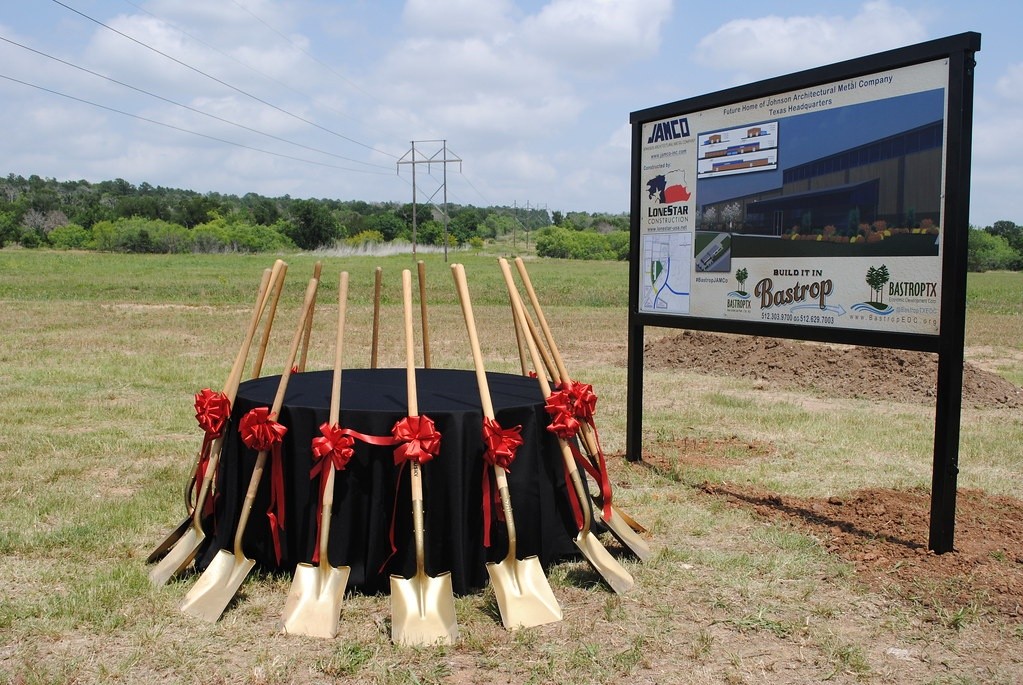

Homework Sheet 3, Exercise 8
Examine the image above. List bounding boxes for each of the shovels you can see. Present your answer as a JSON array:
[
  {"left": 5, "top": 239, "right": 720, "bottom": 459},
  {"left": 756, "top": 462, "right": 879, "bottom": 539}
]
[{"left": 143, "top": 254, "right": 654, "bottom": 649}]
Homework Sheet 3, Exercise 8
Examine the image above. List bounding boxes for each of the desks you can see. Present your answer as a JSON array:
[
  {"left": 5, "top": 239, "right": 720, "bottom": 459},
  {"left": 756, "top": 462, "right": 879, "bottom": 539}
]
[{"left": 196, "top": 368, "right": 608, "bottom": 598}]
